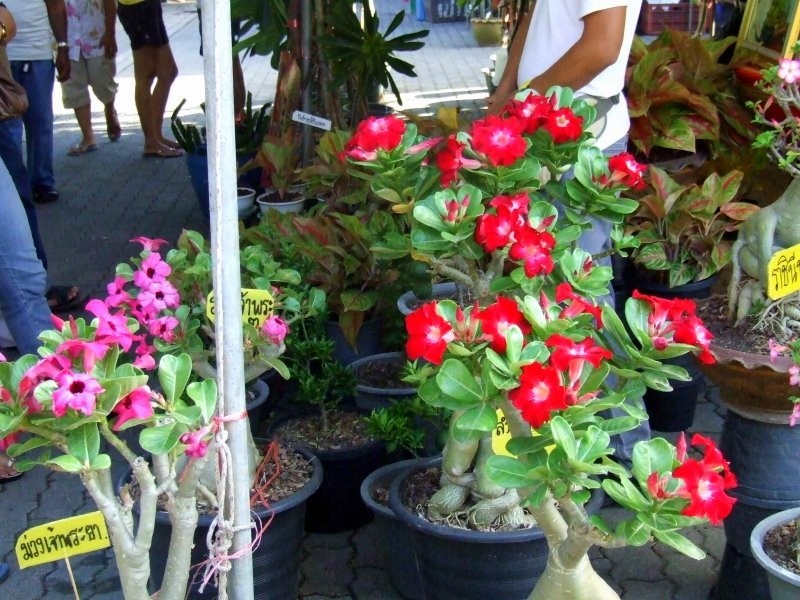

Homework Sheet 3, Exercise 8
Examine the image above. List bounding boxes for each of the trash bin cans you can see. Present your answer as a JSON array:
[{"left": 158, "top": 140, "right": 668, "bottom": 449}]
[
  {"left": 415, "top": 0, "right": 426, "bottom": 21},
  {"left": 410, "top": 0, "right": 416, "bottom": 14},
  {"left": 424, "top": 0, "right": 464, "bottom": 22}
]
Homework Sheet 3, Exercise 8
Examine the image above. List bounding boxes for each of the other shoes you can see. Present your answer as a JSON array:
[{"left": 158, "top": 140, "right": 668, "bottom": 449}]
[
  {"left": 32, "top": 185, "right": 59, "bottom": 201},
  {"left": 596, "top": 470, "right": 646, "bottom": 508}
]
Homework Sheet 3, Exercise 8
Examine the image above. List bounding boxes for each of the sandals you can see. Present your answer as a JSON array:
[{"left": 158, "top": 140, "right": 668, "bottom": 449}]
[{"left": 44, "top": 284, "right": 91, "bottom": 312}]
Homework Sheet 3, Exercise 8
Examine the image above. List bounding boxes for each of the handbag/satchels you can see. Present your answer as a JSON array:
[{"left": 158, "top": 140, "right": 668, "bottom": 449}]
[{"left": 0, "top": 22, "right": 30, "bottom": 120}]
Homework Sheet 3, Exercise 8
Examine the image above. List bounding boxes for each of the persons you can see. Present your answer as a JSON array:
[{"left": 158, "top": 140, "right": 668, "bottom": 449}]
[
  {"left": 197, "top": 0, "right": 247, "bottom": 125},
  {"left": 483, "top": 0, "right": 651, "bottom": 510},
  {"left": 0, "top": 0, "right": 186, "bottom": 481}
]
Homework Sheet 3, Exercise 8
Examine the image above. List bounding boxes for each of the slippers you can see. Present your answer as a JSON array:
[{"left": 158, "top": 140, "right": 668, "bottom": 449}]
[
  {"left": 104, "top": 108, "right": 122, "bottom": 142},
  {"left": 164, "top": 140, "right": 182, "bottom": 148},
  {"left": 69, "top": 141, "right": 99, "bottom": 156},
  {"left": 145, "top": 146, "right": 184, "bottom": 158}
]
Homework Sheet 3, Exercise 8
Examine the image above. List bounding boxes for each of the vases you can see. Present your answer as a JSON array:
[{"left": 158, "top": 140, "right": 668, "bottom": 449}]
[
  {"left": 113, "top": 436, "right": 324, "bottom": 600},
  {"left": 238, "top": 187, "right": 256, "bottom": 212},
  {"left": 391, "top": 457, "right": 605, "bottom": 600},
  {"left": 347, "top": 352, "right": 440, "bottom": 455},
  {"left": 397, "top": 282, "right": 466, "bottom": 316},
  {"left": 621, "top": 256, "right": 716, "bottom": 432},
  {"left": 256, "top": 193, "right": 306, "bottom": 216},
  {"left": 688, "top": 295, "right": 800, "bottom": 408},
  {"left": 286, "top": 181, "right": 309, "bottom": 194},
  {"left": 360, "top": 459, "right": 422, "bottom": 569},
  {"left": 342, "top": 103, "right": 394, "bottom": 127},
  {"left": 187, "top": 145, "right": 263, "bottom": 223},
  {"left": 324, "top": 310, "right": 379, "bottom": 364},
  {"left": 471, "top": 17, "right": 503, "bottom": 47},
  {"left": 154, "top": 377, "right": 269, "bottom": 437},
  {"left": 750, "top": 508, "right": 800, "bottom": 600},
  {"left": 270, "top": 411, "right": 381, "bottom": 535}
]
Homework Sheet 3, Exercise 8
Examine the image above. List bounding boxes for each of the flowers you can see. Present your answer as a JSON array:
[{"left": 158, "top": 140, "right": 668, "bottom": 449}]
[{"left": 0, "top": 0, "right": 800, "bottom": 600}]
